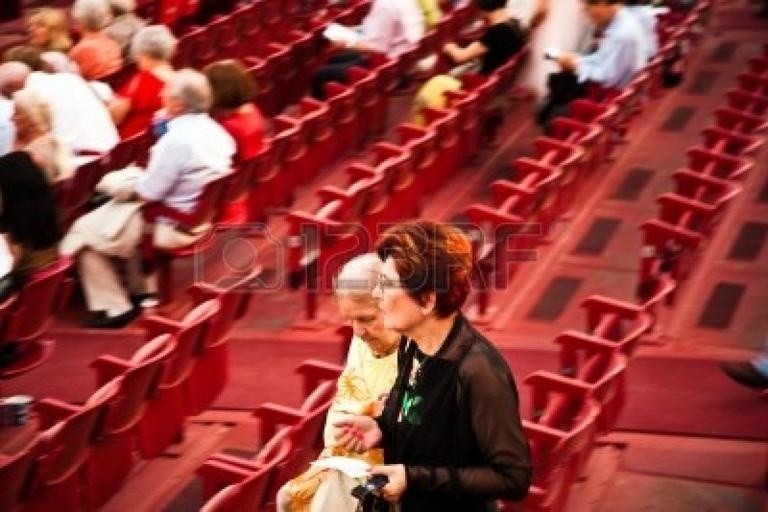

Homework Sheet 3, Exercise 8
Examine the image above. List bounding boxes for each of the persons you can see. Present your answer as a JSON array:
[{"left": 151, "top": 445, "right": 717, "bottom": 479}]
[
  {"left": 331, "top": 219, "right": 532, "bottom": 512},
  {"left": 277, "top": 252, "right": 426, "bottom": 512},
  {"left": 720, "top": 341, "right": 767, "bottom": 393}
]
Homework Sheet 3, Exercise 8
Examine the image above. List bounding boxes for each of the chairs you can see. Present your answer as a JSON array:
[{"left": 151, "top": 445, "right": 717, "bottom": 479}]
[{"left": 0, "top": 1, "right": 765, "bottom": 512}]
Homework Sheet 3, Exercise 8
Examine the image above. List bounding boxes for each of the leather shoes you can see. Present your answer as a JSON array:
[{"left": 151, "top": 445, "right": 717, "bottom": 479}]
[
  {"left": 85, "top": 304, "right": 140, "bottom": 329},
  {"left": 717, "top": 359, "right": 768, "bottom": 389}
]
